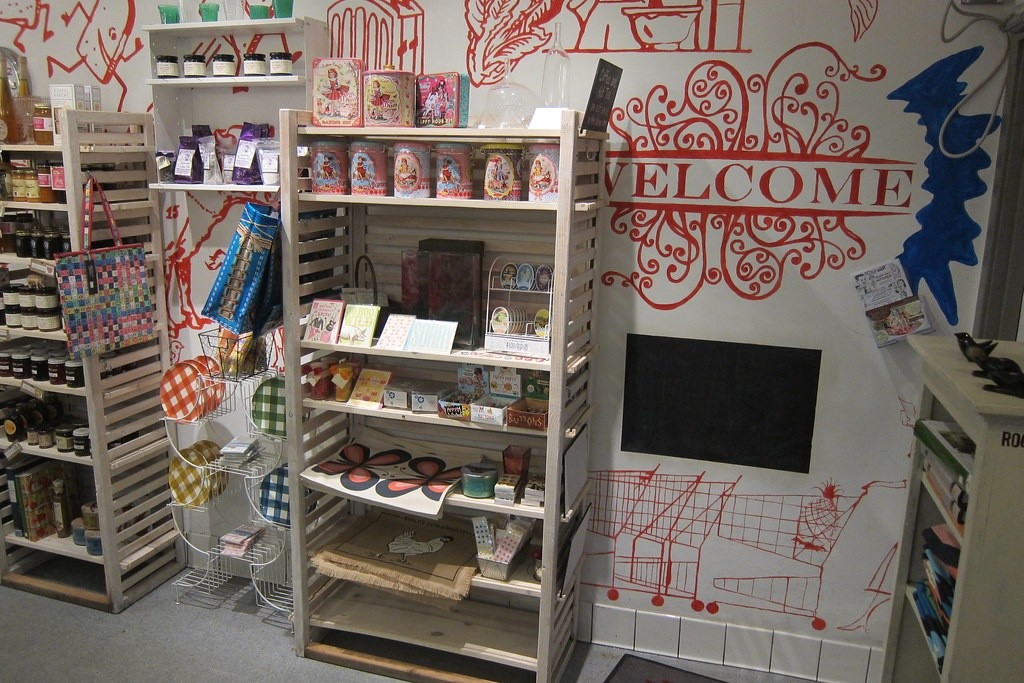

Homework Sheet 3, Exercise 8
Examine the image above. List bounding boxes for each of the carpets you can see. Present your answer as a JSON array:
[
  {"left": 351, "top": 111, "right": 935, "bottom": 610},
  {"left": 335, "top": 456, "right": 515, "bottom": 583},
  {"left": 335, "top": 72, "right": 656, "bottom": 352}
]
[{"left": 603, "top": 654, "right": 729, "bottom": 683}]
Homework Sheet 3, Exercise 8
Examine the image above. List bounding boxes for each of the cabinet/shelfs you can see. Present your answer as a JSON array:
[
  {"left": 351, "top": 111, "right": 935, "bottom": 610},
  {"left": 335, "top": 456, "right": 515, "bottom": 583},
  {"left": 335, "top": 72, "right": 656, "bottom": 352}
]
[
  {"left": 279, "top": 109, "right": 607, "bottom": 683},
  {"left": 880, "top": 335, "right": 1024, "bottom": 683},
  {"left": 141, "top": 16, "right": 327, "bottom": 195},
  {"left": 160, "top": 327, "right": 294, "bottom": 620},
  {"left": 0, "top": 109, "right": 184, "bottom": 613}
]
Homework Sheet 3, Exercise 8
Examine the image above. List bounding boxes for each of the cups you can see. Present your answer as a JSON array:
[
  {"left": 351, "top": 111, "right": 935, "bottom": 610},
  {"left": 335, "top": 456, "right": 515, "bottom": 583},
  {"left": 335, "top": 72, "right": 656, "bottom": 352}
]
[
  {"left": 158, "top": 4, "right": 179, "bottom": 23},
  {"left": 272, "top": 0, "right": 293, "bottom": 18},
  {"left": 199, "top": 3, "right": 219, "bottom": 22},
  {"left": 248, "top": 5, "right": 270, "bottom": 19}
]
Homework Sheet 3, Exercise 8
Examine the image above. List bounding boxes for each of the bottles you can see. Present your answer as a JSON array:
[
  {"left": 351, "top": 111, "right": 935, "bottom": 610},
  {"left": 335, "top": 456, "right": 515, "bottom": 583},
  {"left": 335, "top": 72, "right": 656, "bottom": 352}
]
[
  {"left": 52, "top": 480, "right": 74, "bottom": 539},
  {"left": 542, "top": 23, "right": 571, "bottom": 109}
]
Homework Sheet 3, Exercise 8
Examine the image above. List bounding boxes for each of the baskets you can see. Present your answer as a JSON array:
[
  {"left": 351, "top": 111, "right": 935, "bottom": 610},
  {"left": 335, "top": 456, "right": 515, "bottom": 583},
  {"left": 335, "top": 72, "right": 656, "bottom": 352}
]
[
  {"left": 199, "top": 328, "right": 276, "bottom": 383},
  {"left": 476, "top": 537, "right": 532, "bottom": 582},
  {"left": 354, "top": 255, "right": 401, "bottom": 339}
]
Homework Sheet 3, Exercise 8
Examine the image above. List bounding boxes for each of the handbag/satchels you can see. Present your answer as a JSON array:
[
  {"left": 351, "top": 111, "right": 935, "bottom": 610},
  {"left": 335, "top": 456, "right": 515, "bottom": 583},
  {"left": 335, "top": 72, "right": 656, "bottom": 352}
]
[
  {"left": 53, "top": 177, "right": 154, "bottom": 361},
  {"left": 201, "top": 152, "right": 336, "bottom": 340}
]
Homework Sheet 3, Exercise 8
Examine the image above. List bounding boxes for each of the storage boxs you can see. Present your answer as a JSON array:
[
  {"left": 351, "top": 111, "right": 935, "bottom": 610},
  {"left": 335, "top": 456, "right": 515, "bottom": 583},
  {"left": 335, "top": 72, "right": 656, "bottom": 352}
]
[
  {"left": 417, "top": 72, "right": 470, "bottom": 128},
  {"left": 313, "top": 58, "right": 366, "bottom": 127},
  {"left": 48, "top": 84, "right": 104, "bottom": 146},
  {"left": 411, "top": 383, "right": 457, "bottom": 414},
  {"left": 439, "top": 390, "right": 485, "bottom": 421},
  {"left": 383, "top": 380, "right": 435, "bottom": 410},
  {"left": 506, "top": 397, "right": 549, "bottom": 431},
  {"left": 471, "top": 395, "right": 515, "bottom": 426}
]
